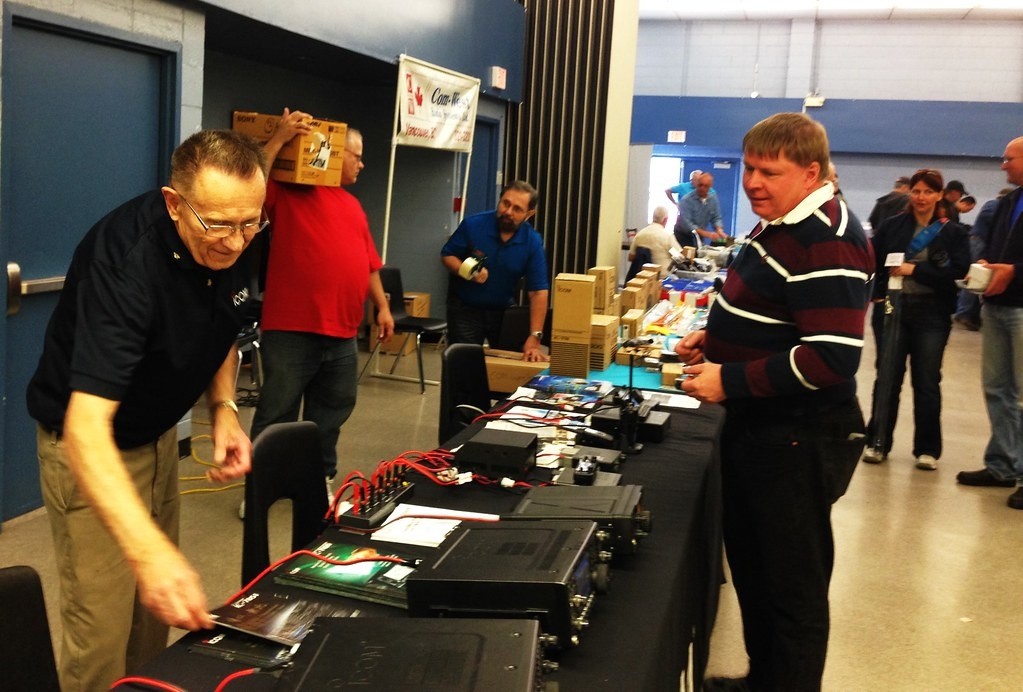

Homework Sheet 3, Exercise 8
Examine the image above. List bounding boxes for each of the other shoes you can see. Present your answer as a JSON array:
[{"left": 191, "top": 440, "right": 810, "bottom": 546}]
[
  {"left": 1008, "top": 485, "right": 1023, "bottom": 509},
  {"left": 328, "top": 477, "right": 351, "bottom": 518},
  {"left": 864, "top": 446, "right": 885, "bottom": 464},
  {"left": 916, "top": 455, "right": 937, "bottom": 470},
  {"left": 237, "top": 500, "right": 243, "bottom": 520},
  {"left": 957, "top": 467, "right": 1017, "bottom": 487},
  {"left": 955, "top": 312, "right": 980, "bottom": 332}
]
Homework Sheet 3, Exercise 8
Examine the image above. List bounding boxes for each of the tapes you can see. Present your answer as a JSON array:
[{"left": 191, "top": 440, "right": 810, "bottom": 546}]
[{"left": 456, "top": 257, "right": 479, "bottom": 280}]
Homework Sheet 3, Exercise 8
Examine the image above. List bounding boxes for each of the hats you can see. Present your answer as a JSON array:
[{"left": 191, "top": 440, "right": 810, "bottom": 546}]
[
  {"left": 961, "top": 193, "right": 977, "bottom": 205},
  {"left": 896, "top": 175, "right": 911, "bottom": 185}
]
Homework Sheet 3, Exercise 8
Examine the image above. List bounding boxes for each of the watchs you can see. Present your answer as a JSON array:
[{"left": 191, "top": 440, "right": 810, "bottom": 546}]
[{"left": 530, "top": 331, "right": 543, "bottom": 339}]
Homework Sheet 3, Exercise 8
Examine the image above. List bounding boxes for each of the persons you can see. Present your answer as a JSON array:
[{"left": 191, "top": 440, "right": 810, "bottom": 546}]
[
  {"left": 665, "top": 169, "right": 716, "bottom": 210},
  {"left": 236, "top": 107, "right": 394, "bottom": 519},
  {"left": 439, "top": 180, "right": 552, "bottom": 362},
  {"left": 955, "top": 135, "right": 1023, "bottom": 510},
  {"left": 24, "top": 128, "right": 267, "bottom": 692},
  {"left": 862, "top": 169, "right": 970, "bottom": 471},
  {"left": 673, "top": 171, "right": 728, "bottom": 248},
  {"left": 675, "top": 112, "right": 877, "bottom": 692},
  {"left": 627, "top": 205, "right": 683, "bottom": 280},
  {"left": 867, "top": 176, "right": 1013, "bottom": 331}
]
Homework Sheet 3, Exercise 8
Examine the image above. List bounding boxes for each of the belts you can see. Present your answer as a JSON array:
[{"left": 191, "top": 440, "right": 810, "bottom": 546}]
[{"left": 38, "top": 422, "right": 60, "bottom": 438}]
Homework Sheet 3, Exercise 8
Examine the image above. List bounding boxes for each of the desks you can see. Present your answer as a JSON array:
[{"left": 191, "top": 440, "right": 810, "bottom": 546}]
[{"left": 107, "top": 269, "right": 728, "bottom": 692}]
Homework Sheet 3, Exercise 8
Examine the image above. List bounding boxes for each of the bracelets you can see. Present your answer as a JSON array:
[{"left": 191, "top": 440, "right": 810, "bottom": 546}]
[{"left": 209, "top": 398, "right": 239, "bottom": 415}]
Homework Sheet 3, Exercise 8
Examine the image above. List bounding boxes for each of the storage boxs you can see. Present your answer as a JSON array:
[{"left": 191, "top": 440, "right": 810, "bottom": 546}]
[
  {"left": 366, "top": 291, "right": 430, "bottom": 356},
  {"left": 233, "top": 110, "right": 347, "bottom": 186},
  {"left": 483, "top": 246, "right": 695, "bottom": 393}
]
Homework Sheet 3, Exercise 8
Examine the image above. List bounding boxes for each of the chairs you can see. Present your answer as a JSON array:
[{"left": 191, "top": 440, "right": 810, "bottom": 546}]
[
  {"left": 0, "top": 565, "right": 61, "bottom": 692},
  {"left": 240, "top": 421, "right": 330, "bottom": 590},
  {"left": 357, "top": 265, "right": 447, "bottom": 394}
]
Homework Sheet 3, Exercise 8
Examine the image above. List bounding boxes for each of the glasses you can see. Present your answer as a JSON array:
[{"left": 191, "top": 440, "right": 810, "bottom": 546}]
[
  {"left": 181, "top": 191, "right": 271, "bottom": 239},
  {"left": 345, "top": 147, "right": 362, "bottom": 163},
  {"left": 1000, "top": 155, "right": 1023, "bottom": 164}
]
